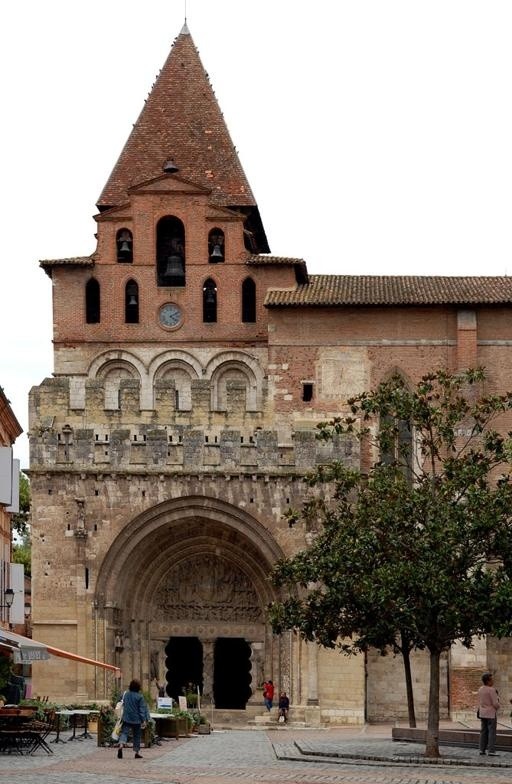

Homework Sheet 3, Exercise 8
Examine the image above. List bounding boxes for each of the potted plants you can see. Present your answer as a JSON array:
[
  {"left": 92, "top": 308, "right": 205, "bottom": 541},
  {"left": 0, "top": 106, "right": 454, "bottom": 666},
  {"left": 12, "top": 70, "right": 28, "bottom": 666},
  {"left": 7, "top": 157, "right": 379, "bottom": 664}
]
[{"left": 178, "top": 690, "right": 213, "bottom": 733}]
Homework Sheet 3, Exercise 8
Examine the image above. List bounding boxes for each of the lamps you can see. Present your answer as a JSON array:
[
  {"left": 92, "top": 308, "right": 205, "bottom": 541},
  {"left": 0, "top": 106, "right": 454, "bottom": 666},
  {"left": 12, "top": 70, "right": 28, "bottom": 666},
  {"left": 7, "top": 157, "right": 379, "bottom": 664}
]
[{"left": 1, "top": 588, "right": 15, "bottom": 607}]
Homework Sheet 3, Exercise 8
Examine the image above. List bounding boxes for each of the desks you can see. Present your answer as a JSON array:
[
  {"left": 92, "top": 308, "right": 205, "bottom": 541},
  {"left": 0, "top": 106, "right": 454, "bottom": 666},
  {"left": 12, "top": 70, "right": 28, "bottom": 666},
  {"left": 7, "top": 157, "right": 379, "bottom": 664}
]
[{"left": 50, "top": 709, "right": 100, "bottom": 744}]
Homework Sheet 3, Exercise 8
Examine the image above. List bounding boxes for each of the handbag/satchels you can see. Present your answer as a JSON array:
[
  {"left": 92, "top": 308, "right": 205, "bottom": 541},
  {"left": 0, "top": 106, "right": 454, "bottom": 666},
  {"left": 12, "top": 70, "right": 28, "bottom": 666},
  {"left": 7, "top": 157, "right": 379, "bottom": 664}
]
[
  {"left": 477, "top": 710, "right": 481, "bottom": 719},
  {"left": 278, "top": 714, "right": 286, "bottom": 722},
  {"left": 115, "top": 702, "right": 124, "bottom": 718}
]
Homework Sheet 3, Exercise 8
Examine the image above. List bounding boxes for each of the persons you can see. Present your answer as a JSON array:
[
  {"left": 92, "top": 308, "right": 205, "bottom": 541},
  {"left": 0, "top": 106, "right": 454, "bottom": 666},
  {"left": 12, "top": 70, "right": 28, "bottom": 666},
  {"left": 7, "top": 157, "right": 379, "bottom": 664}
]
[
  {"left": 118, "top": 679, "right": 152, "bottom": 758},
  {"left": 278, "top": 692, "right": 289, "bottom": 724},
  {"left": 477, "top": 674, "right": 501, "bottom": 756},
  {"left": 262, "top": 681, "right": 274, "bottom": 710}
]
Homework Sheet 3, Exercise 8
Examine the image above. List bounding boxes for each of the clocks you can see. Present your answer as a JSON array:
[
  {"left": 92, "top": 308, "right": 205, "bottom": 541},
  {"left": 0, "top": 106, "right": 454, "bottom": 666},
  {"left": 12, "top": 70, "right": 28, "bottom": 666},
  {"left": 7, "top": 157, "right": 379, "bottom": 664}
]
[{"left": 156, "top": 301, "right": 185, "bottom": 331}]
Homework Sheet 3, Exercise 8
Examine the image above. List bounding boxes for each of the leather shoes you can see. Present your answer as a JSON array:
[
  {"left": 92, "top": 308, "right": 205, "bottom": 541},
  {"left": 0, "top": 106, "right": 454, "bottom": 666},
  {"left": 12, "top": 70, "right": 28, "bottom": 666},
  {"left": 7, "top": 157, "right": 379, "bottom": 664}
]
[{"left": 480, "top": 751, "right": 500, "bottom": 756}]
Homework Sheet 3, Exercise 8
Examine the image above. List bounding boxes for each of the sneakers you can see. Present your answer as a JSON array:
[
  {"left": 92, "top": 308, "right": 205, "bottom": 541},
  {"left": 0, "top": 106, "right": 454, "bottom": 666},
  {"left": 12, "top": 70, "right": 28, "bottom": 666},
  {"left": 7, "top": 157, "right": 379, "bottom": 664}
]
[
  {"left": 135, "top": 754, "right": 143, "bottom": 758},
  {"left": 118, "top": 748, "right": 122, "bottom": 759}
]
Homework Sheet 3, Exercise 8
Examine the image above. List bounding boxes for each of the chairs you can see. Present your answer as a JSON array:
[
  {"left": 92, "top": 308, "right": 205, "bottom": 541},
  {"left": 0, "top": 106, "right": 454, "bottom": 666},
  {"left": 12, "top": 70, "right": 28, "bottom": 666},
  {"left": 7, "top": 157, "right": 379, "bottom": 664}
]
[{"left": 0, "top": 705, "right": 55, "bottom": 755}]
